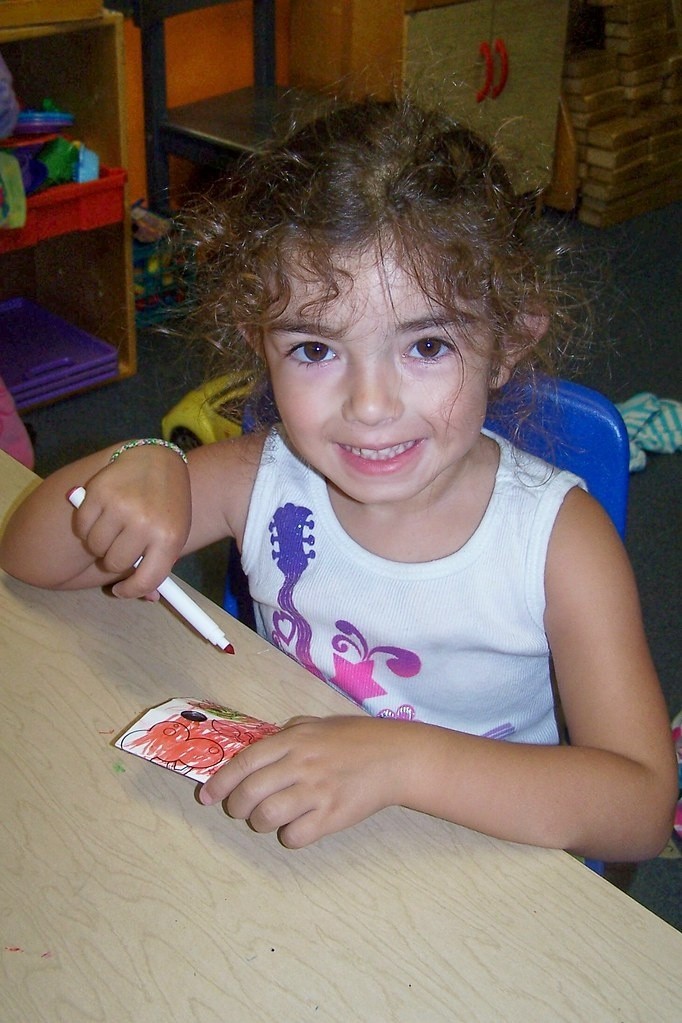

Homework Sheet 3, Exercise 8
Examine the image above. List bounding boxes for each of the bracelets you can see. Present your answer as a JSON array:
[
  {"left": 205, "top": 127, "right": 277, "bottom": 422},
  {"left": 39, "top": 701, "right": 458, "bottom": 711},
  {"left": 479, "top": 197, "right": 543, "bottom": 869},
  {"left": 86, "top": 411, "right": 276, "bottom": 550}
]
[{"left": 109, "top": 438, "right": 189, "bottom": 465}]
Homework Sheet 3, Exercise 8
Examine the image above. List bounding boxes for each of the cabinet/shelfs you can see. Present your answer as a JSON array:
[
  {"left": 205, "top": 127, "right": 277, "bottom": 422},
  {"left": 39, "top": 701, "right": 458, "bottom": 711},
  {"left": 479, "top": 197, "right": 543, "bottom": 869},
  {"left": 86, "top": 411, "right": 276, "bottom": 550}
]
[{"left": 0, "top": 0, "right": 567, "bottom": 353}]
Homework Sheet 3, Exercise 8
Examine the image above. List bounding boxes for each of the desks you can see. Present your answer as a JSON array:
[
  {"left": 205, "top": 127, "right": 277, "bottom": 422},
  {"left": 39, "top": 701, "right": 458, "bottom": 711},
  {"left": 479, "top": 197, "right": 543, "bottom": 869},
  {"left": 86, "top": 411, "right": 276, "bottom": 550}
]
[{"left": 0, "top": 444, "right": 682, "bottom": 1023}]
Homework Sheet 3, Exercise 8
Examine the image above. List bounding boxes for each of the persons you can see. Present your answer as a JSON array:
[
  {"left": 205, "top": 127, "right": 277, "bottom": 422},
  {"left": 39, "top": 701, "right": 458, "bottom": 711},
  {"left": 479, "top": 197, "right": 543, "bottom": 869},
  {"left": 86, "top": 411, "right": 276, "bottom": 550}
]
[{"left": 1, "top": 100, "right": 678, "bottom": 862}]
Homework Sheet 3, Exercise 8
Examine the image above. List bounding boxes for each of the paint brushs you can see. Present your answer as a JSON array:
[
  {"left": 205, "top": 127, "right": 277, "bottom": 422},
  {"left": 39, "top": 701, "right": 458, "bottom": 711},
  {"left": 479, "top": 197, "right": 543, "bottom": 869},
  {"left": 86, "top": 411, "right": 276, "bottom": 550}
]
[{"left": 58, "top": 482, "right": 245, "bottom": 657}]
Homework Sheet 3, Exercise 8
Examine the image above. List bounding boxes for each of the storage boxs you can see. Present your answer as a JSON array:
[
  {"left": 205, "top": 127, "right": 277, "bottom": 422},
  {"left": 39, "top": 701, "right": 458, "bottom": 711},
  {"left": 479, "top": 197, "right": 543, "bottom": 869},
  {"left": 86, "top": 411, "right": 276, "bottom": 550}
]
[{"left": 0, "top": 166, "right": 131, "bottom": 253}]
